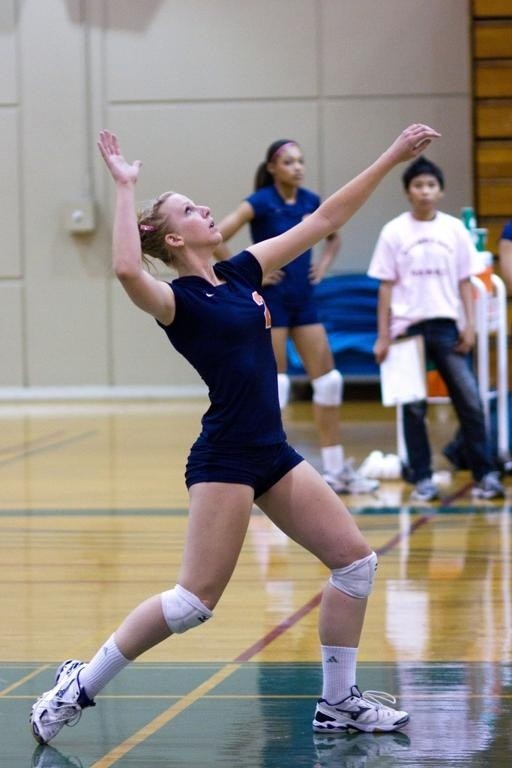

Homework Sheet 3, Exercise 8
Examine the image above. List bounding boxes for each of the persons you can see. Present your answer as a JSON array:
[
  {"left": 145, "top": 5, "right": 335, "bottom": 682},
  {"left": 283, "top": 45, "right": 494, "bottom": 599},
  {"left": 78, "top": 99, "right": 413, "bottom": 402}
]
[
  {"left": 443, "top": 203, "right": 511, "bottom": 469},
  {"left": 372, "top": 155, "right": 507, "bottom": 504},
  {"left": 30, "top": 121, "right": 443, "bottom": 749},
  {"left": 496, "top": 222, "right": 511, "bottom": 297},
  {"left": 209, "top": 134, "right": 381, "bottom": 498}
]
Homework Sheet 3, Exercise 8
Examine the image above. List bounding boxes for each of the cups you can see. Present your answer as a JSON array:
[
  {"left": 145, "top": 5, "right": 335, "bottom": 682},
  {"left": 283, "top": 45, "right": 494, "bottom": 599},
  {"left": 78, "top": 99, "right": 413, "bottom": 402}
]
[{"left": 461, "top": 206, "right": 487, "bottom": 253}]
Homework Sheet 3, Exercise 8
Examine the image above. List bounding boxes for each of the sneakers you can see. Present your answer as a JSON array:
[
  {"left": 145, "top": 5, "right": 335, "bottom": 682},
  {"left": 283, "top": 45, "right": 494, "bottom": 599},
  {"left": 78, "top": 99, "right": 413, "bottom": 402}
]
[
  {"left": 312, "top": 685, "right": 408, "bottom": 733},
  {"left": 30, "top": 659, "right": 96, "bottom": 745},
  {"left": 409, "top": 465, "right": 504, "bottom": 501},
  {"left": 319, "top": 458, "right": 380, "bottom": 495}
]
